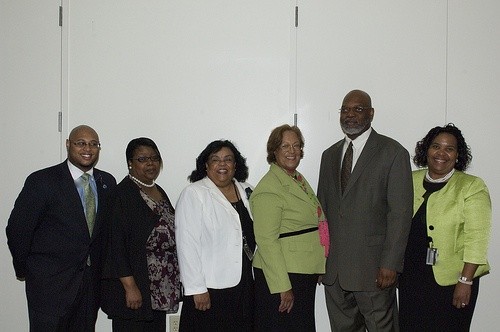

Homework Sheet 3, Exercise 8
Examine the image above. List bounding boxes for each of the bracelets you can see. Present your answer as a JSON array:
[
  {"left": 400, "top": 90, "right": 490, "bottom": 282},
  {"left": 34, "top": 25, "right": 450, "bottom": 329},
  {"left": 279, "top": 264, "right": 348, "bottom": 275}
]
[{"left": 457, "top": 277, "right": 473, "bottom": 286}]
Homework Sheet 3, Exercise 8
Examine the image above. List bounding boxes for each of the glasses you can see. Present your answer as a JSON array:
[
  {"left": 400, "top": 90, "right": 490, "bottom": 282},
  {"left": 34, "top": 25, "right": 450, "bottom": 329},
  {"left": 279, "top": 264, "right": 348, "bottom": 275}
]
[
  {"left": 279, "top": 143, "right": 303, "bottom": 151},
  {"left": 338, "top": 107, "right": 369, "bottom": 113},
  {"left": 70, "top": 140, "right": 101, "bottom": 149},
  {"left": 131, "top": 156, "right": 162, "bottom": 163}
]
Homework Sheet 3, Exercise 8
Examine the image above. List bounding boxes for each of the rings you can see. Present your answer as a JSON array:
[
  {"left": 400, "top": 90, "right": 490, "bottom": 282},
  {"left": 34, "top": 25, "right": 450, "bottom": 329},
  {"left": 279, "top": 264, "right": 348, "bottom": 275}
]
[{"left": 461, "top": 303, "right": 466, "bottom": 306}]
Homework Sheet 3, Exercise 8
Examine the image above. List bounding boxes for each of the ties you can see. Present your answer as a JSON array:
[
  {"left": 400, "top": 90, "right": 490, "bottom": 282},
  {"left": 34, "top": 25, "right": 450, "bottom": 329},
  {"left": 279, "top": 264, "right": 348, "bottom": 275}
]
[
  {"left": 341, "top": 141, "right": 353, "bottom": 195},
  {"left": 80, "top": 173, "right": 97, "bottom": 267}
]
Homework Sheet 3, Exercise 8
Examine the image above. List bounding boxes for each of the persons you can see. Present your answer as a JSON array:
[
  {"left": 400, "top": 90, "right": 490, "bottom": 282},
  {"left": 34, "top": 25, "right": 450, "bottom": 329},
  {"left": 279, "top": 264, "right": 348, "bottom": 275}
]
[
  {"left": 97, "top": 137, "right": 185, "bottom": 332},
  {"left": 174, "top": 140, "right": 257, "bottom": 332},
  {"left": 249, "top": 123, "right": 331, "bottom": 332},
  {"left": 317, "top": 90, "right": 414, "bottom": 332},
  {"left": 6, "top": 125, "right": 117, "bottom": 332},
  {"left": 396, "top": 122, "right": 492, "bottom": 332}
]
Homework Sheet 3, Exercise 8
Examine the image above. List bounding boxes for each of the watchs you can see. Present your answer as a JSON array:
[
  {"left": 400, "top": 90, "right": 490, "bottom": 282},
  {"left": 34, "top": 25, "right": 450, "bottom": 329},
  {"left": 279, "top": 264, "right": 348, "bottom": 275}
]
[{"left": 459, "top": 276, "right": 472, "bottom": 282}]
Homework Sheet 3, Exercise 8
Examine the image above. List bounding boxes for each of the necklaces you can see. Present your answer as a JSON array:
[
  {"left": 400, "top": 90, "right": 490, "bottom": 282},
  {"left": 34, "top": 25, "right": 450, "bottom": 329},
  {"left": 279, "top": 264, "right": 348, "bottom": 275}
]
[
  {"left": 128, "top": 173, "right": 155, "bottom": 188},
  {"left": 426, "top": 167, "right": 455, "bottom": 183}
]
